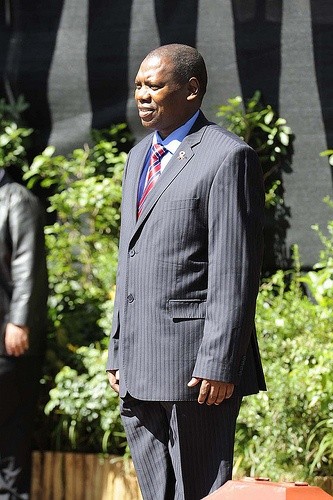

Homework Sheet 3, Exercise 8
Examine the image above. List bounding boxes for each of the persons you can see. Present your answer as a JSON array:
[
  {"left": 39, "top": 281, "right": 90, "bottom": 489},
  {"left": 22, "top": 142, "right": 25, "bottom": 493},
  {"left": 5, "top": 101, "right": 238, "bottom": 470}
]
[
  {"left": 0, "top": 170, "right": 53, "bottom": 500},
  {"left": 102, "top": 43, "right": 268, "bottom": 500}
]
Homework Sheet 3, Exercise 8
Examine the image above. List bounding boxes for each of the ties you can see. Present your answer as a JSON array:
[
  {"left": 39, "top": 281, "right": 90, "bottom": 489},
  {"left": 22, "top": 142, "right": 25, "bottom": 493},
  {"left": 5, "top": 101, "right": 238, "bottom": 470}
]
[{"left": 137, "top": 143, "right": 168, "bottom": 219}]
